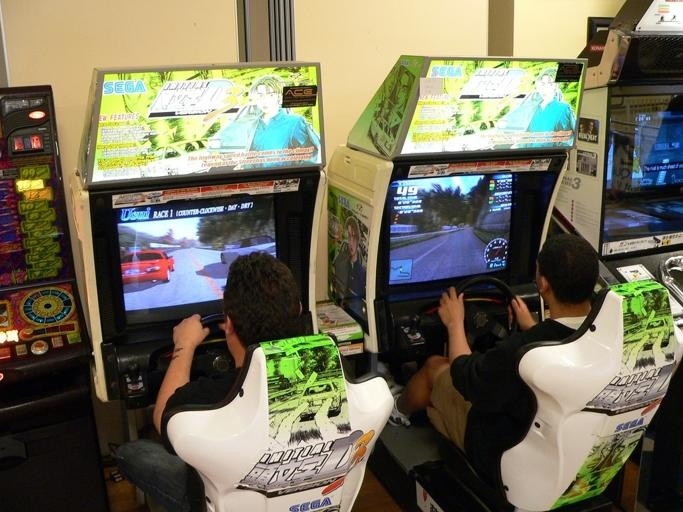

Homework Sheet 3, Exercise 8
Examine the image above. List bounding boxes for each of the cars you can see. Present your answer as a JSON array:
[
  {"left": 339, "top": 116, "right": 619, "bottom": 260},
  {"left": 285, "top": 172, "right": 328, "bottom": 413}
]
[
  {"left": 443, "top": 119, "right": 509, "bottom": 153},
  {"left": 217, "top": 234, "right": 277, "bottom": 266},
  {"left": 121, "top": 249, "right": 174, "bottom": 287},
  {"left": 137, "top": 137, "right": 213, "bottom": 178}
]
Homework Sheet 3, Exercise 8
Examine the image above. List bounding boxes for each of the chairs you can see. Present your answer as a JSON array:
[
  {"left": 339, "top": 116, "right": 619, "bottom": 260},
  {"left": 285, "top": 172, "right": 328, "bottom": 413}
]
[
  {"left": 162, "top": 332, "right": 398, "bottom": 512},
  {"left": 438, "top": 278, "right": 681, "bottom": 510}
]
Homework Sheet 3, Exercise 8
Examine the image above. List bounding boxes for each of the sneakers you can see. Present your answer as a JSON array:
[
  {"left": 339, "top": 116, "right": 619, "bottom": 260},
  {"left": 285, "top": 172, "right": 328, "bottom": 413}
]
[{"left": 378, "top": 360, "right": 412, "bottom": 428}]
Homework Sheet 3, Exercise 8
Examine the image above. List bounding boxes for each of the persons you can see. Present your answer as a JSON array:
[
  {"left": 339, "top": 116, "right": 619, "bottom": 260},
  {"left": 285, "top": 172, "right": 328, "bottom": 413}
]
[
  {"left": 112, "top": 250, "right": 306, "bottom": 512},
  {"left": 377, "top": 233, "right": 599, "bottom": 477}
]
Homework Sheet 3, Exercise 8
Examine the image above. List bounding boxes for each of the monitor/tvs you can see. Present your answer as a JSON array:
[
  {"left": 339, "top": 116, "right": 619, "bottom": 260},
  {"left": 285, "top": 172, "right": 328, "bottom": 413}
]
[
  {"left": 600, "top": 94, "right": 683, "bottom": 242},
  {"left": 387, "top": 172, "right": 516, "bottom": 285},
  {"left": 113, "top": 195, "right": 279, "bottom": 331}
]
